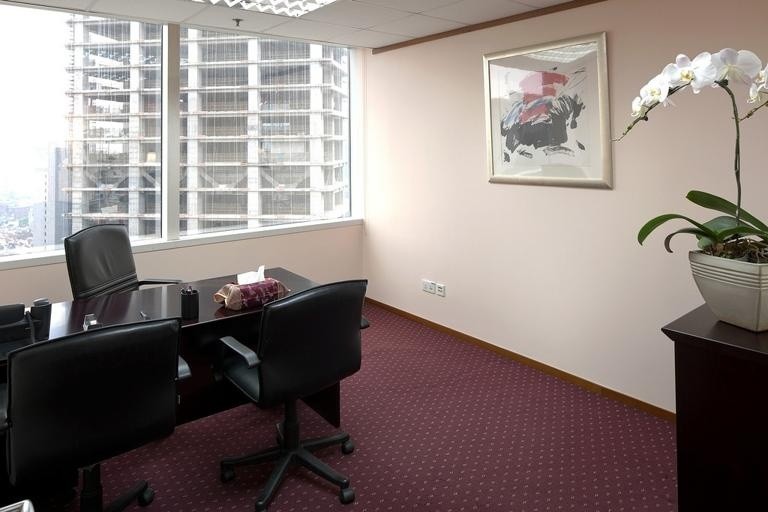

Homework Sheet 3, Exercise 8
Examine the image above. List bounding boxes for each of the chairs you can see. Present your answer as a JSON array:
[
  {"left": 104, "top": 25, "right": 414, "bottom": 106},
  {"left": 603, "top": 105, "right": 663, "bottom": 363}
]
[{"left": 63, "top": 223, "right": 183, "bottom": 300}]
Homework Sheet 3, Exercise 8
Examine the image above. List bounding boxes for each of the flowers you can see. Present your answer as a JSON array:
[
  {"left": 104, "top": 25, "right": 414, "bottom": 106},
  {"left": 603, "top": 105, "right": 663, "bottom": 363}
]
[{"left": 610, "top": 48, "right": 768, "bottom": 255}]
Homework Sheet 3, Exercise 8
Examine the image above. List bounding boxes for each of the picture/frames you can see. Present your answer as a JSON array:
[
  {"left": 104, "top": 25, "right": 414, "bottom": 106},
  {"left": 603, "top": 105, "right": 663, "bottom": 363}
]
[{"left": 482, "top": 31, "right": 615, "bottom": 190}]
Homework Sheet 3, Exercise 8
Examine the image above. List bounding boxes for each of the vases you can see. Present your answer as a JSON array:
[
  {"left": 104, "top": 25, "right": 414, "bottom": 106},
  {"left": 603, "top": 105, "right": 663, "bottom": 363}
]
[{"left": 688, "top": 250, "right": 768, "bottom": 334}]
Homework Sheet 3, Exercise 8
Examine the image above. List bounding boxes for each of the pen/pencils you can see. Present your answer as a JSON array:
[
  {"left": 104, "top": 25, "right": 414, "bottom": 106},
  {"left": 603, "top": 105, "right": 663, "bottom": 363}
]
[
  {"left": 140, "top": 311, "right": 148, "bottom": 318},
  {"left": 181, "top": 285, "right": 193, "bottom": 295}
]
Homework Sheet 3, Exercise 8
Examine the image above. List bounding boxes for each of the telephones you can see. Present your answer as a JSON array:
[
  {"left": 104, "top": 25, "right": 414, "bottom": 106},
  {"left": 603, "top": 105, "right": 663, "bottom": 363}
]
[{"left": 0, "top": 305, "right": 40, "bottom": 344}]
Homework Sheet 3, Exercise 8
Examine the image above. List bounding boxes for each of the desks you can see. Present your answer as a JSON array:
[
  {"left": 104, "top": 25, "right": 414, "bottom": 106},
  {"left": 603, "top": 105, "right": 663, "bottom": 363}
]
[{"left": 0, "top": 267, "right": 341, "bottom": 429}]
